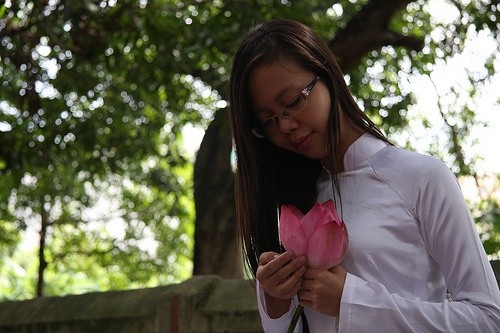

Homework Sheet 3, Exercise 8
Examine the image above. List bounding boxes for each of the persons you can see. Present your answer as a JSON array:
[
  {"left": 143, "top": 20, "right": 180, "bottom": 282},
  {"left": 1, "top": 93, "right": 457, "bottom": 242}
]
[{"left": 229, "top": 17, "right": 500, "bottom": 333}]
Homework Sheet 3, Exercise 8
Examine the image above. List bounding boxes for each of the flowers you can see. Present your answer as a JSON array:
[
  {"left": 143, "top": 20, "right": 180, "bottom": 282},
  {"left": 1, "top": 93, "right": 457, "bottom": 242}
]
[{"left": 279, "top": 198, "right": 349, "bottom": 333}]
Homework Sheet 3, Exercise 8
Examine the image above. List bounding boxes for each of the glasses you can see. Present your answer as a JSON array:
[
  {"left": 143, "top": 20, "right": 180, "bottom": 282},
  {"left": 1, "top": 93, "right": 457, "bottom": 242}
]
[{"left": 251, "top": 73, "right": 323, "bottom": 139}]
